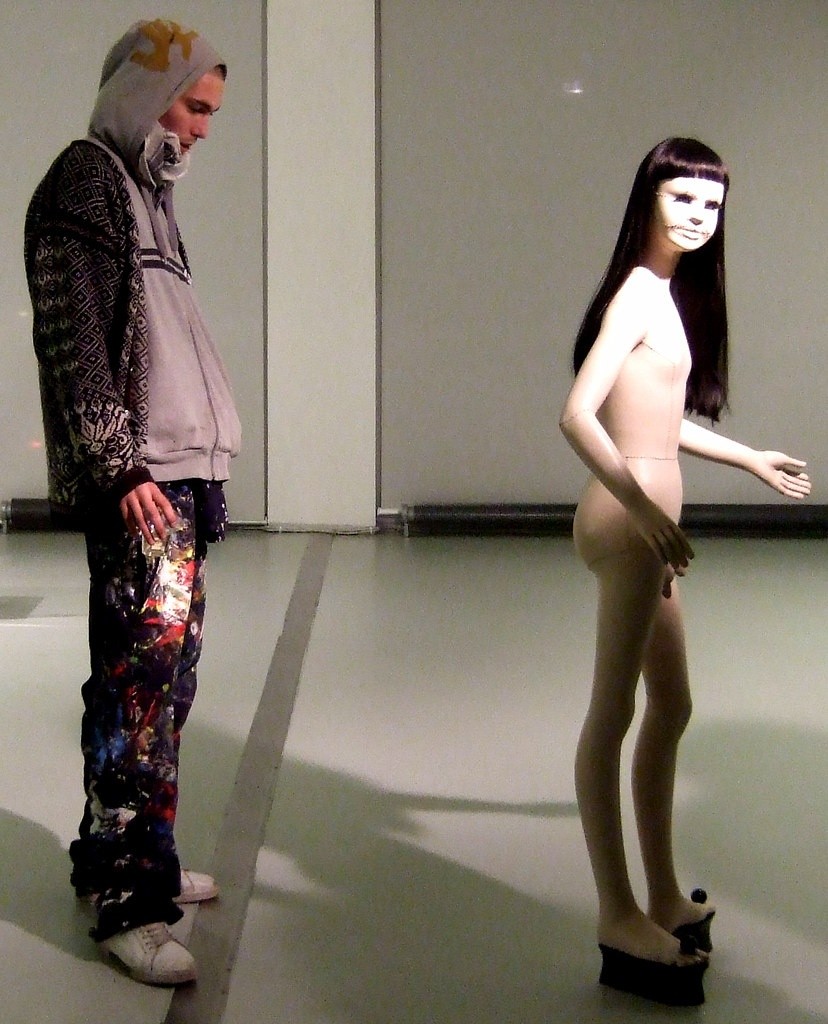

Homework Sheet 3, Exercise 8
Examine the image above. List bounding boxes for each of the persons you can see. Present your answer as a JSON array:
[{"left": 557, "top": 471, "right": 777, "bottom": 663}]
[
  {"left": 561, "top": 140, "right": 811, "bottom": 1010},
  {"left": 23, "top": 15, "right": 243, "bottom": 985}
]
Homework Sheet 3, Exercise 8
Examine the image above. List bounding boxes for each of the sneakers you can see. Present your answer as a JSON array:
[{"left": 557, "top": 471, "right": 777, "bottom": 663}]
[
  {"left": 96, "top": 921, "right": 196, "bottom": 983},
  {"left": 88, "top": 868, "right": 220, "bottom": 903}
]
